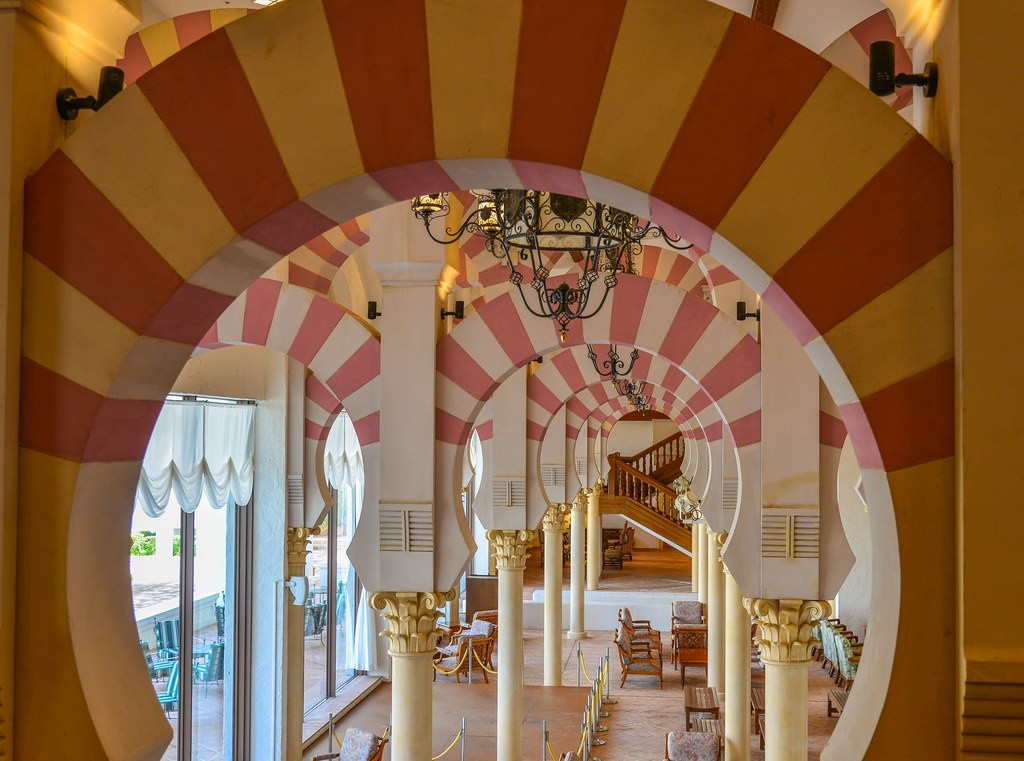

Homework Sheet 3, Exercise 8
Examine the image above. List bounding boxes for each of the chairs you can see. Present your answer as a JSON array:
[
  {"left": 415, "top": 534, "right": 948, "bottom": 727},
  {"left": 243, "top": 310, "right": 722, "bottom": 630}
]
[
  {"left": 433, "top": 610, "right": 499, "bottom": 686},
  {"left": 303, "top": 580, "right": 346, "bottom": 647},
  {"left": 663, "top": 731, "right": 722, "bottom": 761},
  {"left": 613, "top": 600, "right": 765, "bottom": 690},
  {"left": 139, "top": 590, "right": 227, "bottom": 719},
  {"left": 313, "top": 728, "right": 389, "bottom": 761},
  {"left": 539, "top": 520, "right": 634, "bottom": 580},
  {"left": 811, "top": 617, "right": 864, "bottom": 691}
]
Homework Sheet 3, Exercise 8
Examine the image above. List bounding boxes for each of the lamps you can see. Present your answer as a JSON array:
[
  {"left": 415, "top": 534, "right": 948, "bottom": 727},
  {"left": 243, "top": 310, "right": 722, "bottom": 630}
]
[
  {"left": 737, "top": 302, "right": 760, "bottom": 322},
  {"left": 409, "top": 188, "right": 696, "bottom": 417},
  {"left": 368, "top": 301, "right": 381, "bottom": 319},
  {"left": 869, "top": 40, "right": 939, "bottom": 98},
  {"left": 56, "top": 66, "right": 125, "bottom": 120}
]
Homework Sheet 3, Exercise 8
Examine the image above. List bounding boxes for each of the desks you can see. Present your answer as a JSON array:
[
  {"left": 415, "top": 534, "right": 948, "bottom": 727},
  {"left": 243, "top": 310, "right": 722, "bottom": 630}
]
[
  {"left": 311, "top": 588, "right": 327, "bottom": 604},
  {"left": 438, "top": 621, "right": 471, "bottom": 644},
  {"left": 678, "top": 648, "right": 708, "bottom": 690},
  {"left": 161, "top": 644, "right": 209, "bottom": 681}
]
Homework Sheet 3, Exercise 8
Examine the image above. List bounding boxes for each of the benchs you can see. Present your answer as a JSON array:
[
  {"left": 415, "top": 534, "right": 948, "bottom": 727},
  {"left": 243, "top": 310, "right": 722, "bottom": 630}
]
[{"left": 684, "top": 685, "right": 850, "bottom": 761}]
